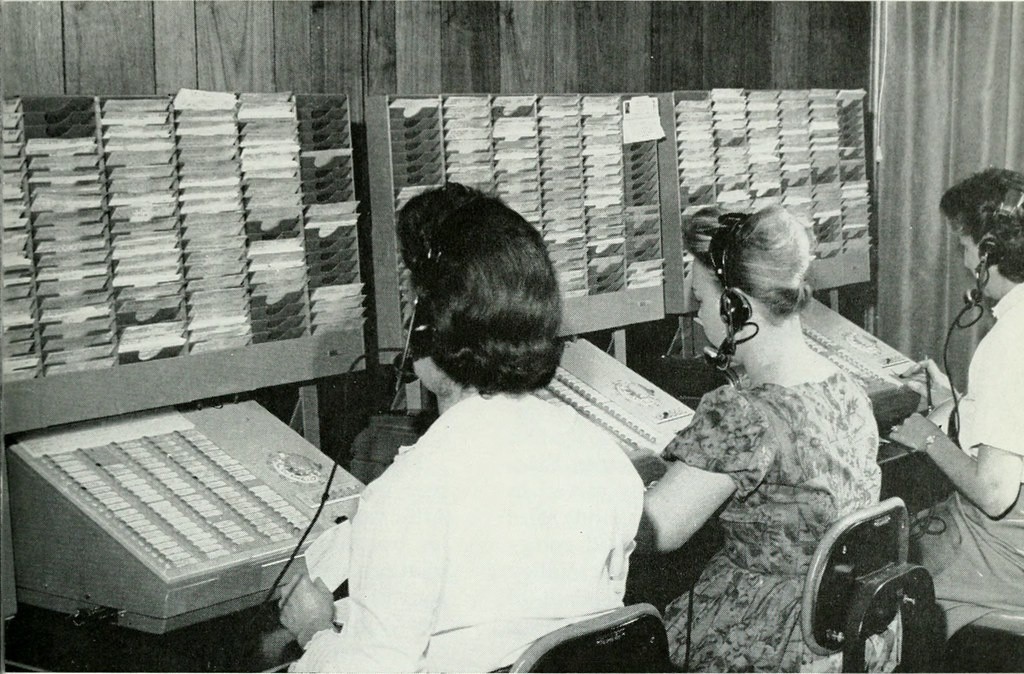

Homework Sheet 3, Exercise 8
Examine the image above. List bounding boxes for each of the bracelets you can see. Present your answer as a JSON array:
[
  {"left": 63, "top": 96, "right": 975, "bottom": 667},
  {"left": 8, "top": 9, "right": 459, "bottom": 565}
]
[{"left": 923, "top": 433, "right": 941, "bottom": 452}]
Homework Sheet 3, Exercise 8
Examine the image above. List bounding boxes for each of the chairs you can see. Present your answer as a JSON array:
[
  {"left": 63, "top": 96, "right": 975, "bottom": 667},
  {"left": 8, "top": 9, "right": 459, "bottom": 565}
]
[
  {"left": 510, "top": 603, "right": 671, "bottom": 673},
  {"left": 799, "top": 498, "right": 949, "bottom": 674}
]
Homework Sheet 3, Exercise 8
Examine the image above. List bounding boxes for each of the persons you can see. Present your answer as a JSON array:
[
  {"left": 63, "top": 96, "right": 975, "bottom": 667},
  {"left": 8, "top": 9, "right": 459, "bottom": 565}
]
[
  {"left": 885, "top": 168, "right": 1024, "bottom": 637},
  {"left": 278, "top": 186, "right": 645, "bottom": 674},
  {"left": 642, "top": 201, "right": 904, "bottom": 673}
]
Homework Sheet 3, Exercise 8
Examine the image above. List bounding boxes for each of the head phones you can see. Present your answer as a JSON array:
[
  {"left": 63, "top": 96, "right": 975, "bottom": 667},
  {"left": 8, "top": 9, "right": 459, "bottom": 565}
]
[
  {"left": 403, "top": 197, "right": 493, "bottom": 353},
  {"left": 719, "top": 212, "right": 754, "bottom": 327},
  {"left": 977, "top": 173, "right": 1024, "bottom": 266}
]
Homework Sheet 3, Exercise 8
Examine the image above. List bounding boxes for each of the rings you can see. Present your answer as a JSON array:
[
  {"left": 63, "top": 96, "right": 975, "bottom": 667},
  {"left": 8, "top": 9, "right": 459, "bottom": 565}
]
[{"left": 890, "top": 426, "right": 899, "bottom": 432}]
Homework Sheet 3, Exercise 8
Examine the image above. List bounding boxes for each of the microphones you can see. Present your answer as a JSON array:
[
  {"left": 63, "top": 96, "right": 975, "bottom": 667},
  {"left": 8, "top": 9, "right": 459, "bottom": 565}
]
[
  {"left": 703, "top": 312, "right": 737, "bottom": 371},
  {"left": 393, "top": 353, "right": 419, "bottom": 392},
  {"left": 965, "top": 243, "right": 997, "bottom": 306}
]
